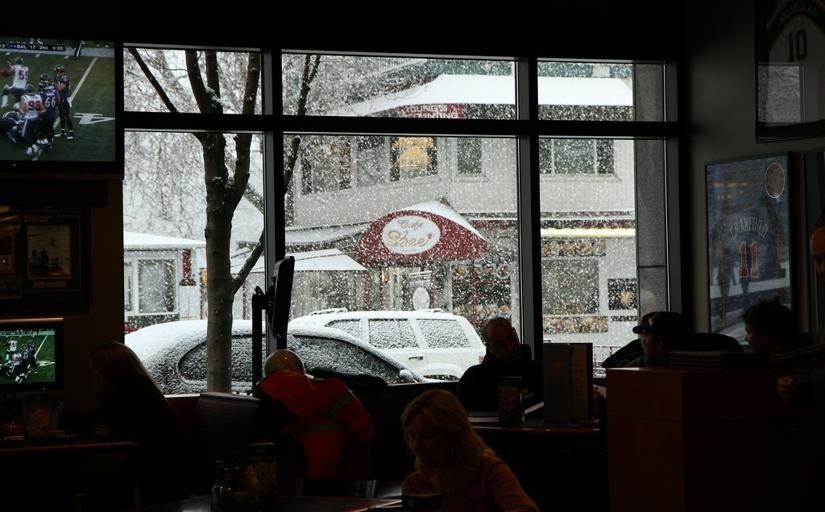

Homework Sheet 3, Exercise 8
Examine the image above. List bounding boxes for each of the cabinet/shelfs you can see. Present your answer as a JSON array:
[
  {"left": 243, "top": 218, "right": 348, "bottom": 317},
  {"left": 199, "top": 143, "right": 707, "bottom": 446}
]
[{"left": 605, "top": 361, "right": 817, "bottom": 512}]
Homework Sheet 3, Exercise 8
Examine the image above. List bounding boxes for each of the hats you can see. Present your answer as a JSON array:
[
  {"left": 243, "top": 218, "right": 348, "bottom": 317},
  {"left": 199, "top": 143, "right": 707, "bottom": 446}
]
[{"left": 632, "top": 310, "right": 683, "bottom": 345}]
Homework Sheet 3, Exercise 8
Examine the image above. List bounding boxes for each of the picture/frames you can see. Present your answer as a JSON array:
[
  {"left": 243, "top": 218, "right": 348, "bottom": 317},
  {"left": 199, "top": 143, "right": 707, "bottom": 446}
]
[{"left": 0, "top": 202, "right": 95, "bottom": 315}]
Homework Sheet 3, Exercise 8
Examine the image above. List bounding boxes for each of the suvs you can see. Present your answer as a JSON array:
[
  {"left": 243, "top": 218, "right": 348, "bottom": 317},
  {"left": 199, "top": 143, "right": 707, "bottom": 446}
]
[{"left": 286, "top": 305, "right": 486, "bottom": 384}]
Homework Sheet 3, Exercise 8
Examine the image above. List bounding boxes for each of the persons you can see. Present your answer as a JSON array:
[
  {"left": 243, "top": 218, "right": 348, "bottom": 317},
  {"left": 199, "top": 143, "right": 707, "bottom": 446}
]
[
  {"left": 450, "top": 318, "right": 599, "bottom": 420},
  {"left": 715, "top": 246, "right": 751, "bottom": 320},
  {"left": 401, "top": 389, "right": 540, "bottom": 512},
  {"left": 0, "top": 337, "right": 40, "bottom": 383},
  {"left": 591, "top": 295, "right": 814, "bottom": 366},
  {"left": 56, "top": 342, "right": 192, "bottom": 510},
  {"left": 0, "top": 56, "right": 74, "bottom": 161},
  {"left": 255, "top": 350, "right": 375, "bottom": 496}
]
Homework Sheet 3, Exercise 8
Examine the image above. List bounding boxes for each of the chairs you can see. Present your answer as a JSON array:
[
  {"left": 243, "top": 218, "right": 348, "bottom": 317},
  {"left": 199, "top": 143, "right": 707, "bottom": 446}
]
[{"left": 176, "top": 387, "right": 279, "bottom": 510}]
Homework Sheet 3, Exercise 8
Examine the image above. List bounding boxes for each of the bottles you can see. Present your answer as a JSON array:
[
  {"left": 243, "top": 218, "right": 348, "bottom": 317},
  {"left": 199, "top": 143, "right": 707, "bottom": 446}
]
[{"left": 209, "top": 459, "right": 261, "bottom": 512}]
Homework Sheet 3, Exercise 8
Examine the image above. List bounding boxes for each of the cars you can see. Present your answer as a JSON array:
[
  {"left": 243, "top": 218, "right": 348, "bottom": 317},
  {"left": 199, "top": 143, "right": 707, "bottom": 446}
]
[{"left": 124, "top": 318, "right": 450, "bottom": 397}]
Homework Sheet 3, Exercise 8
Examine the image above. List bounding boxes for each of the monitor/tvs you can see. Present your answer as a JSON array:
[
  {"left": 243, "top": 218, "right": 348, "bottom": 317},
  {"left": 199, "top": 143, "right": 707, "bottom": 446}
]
[
  {"left": 0, "top": 35, "right": 125, "bottom": 174},
  {"left": 751, "top": 1, "right": 823, "bottom": 142},
  {"left": 0, "top": 330, "right": 57, "bottom": 387},
  {"left": 571, "top": 342, "right": 593, "bottom": 424},
  {"left": 542, "top": 343, "right": 571, "bottom": 420},
  {"left": 269, "top": 256, "right": 296, "bottom": 349}
]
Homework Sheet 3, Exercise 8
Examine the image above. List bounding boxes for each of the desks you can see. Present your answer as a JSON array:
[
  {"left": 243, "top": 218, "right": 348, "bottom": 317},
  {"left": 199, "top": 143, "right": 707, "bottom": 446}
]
[
  {"left": 1, "top": 435, "right": 139, "bottom": 511},
  {"left": 472, "top": 413, "right": 601, "bottom": 437},
  {"left": 163, "top": 488, "right": 402, "bottom": 512}
]
